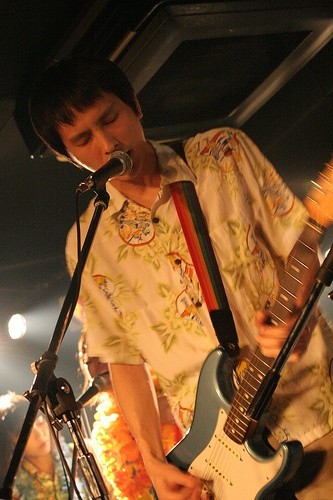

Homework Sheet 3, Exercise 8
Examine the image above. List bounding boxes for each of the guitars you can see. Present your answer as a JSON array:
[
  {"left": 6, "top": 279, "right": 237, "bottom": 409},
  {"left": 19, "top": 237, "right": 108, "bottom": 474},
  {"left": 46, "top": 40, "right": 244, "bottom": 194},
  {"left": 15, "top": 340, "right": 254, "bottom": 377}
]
[{"left": 149, "top": 155, "right": 333, "bottom": 500}]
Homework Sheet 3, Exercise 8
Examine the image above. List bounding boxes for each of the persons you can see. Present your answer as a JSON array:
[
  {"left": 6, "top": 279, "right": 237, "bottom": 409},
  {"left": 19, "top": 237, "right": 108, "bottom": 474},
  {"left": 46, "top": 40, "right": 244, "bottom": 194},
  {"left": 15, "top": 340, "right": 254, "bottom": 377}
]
[
  {"left": 76, "top": 332, "right": 182, "bottom": 500},
  {"left": 0, "top": 391, "right": 94, "bottom": 500},
  {"left": 27, "top": 54, "right": 333, "bottom": 500}
]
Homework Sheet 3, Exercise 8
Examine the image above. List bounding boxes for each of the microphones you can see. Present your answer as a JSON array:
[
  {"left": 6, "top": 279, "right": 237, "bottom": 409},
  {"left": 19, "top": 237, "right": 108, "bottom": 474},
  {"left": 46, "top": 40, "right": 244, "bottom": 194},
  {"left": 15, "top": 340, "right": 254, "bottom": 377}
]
[{"left": 75, "top": 150, "right": 132, "bottom": 197}]
